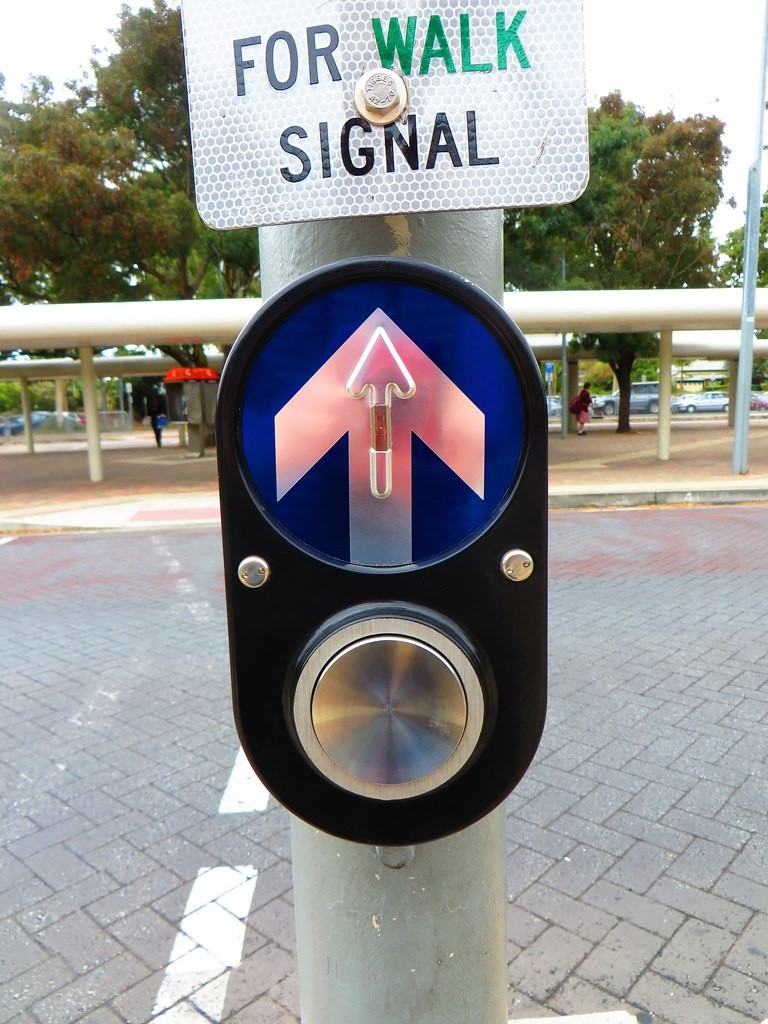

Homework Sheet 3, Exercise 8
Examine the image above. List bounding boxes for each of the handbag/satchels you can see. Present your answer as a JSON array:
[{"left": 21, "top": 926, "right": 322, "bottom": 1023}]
[{"left": 157, "top": 414, "right": 168, "bottom": 429}]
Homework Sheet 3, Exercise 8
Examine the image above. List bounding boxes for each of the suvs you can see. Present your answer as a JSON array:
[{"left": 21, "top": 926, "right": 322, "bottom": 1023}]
[{"left": 590, "top": 382, "right": 659, "bottom": 416}]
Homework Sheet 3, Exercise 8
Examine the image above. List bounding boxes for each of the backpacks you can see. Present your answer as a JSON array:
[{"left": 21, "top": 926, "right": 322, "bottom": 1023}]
[{"left": 569, "top": 392, "right": 588, "bottom": 414}]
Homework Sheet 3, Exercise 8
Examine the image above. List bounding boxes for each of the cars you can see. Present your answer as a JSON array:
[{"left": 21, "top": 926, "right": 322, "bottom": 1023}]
[
  {"left": 547, "top": 395, "right": 562, "bottom": 416},
  {"left": 0, "top": 410, "right": 87, "bottom": 437},
  {"left": 671, "top": 391, "right": 730, "bottom": 413},
  {"left": 751, "top": 392, "right": 768, "bottom": 410}
]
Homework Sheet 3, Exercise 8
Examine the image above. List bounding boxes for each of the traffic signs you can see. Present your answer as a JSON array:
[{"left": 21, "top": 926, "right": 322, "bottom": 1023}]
[{"left": 181, "top": 0, "right": 589, "bottom": 229}]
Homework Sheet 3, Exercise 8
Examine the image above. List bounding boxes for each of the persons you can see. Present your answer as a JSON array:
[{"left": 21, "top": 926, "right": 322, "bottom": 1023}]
[
  {"left": 150, "top": 399, "right": 164, "bottom": 448},
  {"left": 576, "top": 382, "right": 592, "bottom": 436}
]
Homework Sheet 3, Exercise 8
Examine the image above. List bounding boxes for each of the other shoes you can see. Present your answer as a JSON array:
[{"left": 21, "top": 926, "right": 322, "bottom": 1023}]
[
  {"left": 157, "top": 444, "right": 161, "bottom": 448},
  {"left": 578, "top": 431, "right": 587, "bottom": 435}
]
